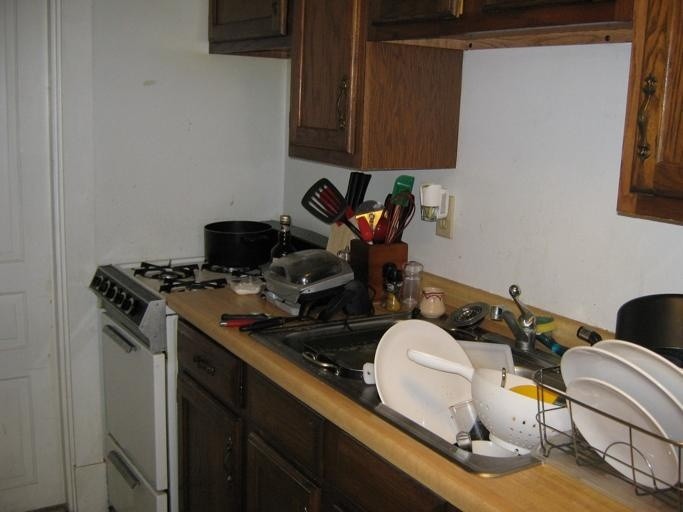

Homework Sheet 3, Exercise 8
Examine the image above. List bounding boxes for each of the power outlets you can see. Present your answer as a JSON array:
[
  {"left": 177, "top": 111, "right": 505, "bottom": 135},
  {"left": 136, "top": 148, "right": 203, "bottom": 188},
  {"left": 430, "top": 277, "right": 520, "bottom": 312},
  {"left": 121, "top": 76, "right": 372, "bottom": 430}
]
[{"left": 435, "top": 197, "right": 456, "bottom": 238}]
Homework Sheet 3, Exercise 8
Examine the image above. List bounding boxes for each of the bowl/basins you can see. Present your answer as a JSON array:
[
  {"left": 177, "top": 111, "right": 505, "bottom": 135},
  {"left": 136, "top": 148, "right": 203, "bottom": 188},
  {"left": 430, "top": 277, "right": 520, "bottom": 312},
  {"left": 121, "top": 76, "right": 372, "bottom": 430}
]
[
  {"left": 471, "top": 440, "right": 515, "bottom": 456},
  {"left": 224, "top": 277, "right": 263, "bottom": 296}
]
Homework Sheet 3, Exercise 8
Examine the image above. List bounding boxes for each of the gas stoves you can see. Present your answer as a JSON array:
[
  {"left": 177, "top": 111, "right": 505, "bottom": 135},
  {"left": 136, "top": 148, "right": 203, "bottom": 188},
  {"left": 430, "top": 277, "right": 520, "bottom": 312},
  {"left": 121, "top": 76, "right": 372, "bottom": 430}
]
[{"left": 110, "top": 255, "right": 265, "bottom": 298}]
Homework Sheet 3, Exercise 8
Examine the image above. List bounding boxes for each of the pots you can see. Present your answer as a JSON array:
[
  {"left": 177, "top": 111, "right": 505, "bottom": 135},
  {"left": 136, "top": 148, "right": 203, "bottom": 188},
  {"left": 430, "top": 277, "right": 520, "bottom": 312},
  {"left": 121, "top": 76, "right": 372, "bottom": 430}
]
[
  {"left": 615, "top": 293, "right": 683, "bottom": 368},
  {"left": 203, "top": 221, "right": 272, "bottom": 267}
]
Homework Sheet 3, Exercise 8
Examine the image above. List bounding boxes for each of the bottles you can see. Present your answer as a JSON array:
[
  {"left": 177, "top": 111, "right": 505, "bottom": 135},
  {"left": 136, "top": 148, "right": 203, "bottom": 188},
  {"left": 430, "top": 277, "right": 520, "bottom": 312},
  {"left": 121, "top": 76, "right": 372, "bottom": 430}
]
[
  {"left": 337, "top": 245, "right": 350, "bottom": 263},
  {"left": 380, "top": 262, "right": 402, "bottom": 312},
  {"left": 403, "top": 261, "right": 423, "bottom": 306},
  {"left": 419, "top": 287, "right": 446, "bottom": 319},
  {"left": 273, "top": 215, "right": 296, "bottom": 258}
]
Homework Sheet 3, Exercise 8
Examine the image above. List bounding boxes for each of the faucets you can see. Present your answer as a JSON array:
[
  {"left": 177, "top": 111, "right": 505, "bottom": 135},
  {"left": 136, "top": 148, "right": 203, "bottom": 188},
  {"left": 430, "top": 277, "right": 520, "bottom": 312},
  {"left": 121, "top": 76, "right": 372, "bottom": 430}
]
[{"left": 490, "top": 304, "right": 534, "bottom": 352}]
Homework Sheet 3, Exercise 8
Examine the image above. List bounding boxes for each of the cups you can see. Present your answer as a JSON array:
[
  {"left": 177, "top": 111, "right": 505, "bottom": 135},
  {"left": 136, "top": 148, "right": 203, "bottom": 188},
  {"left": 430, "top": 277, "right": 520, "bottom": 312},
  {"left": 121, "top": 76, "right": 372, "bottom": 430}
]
[
  {"left": 419, "top": 183, "right": 450, "bottom": 222},
  {"left": 448, "top": 399, "right": 485, "bottom": 440}
]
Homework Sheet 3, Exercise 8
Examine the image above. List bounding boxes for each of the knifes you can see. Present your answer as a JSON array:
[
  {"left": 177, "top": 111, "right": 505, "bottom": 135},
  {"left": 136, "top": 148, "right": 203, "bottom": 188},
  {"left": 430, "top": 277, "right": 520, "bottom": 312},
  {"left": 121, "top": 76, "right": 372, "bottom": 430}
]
[{"left": 341, "top": 172, "right": 372, "bottom": 218}]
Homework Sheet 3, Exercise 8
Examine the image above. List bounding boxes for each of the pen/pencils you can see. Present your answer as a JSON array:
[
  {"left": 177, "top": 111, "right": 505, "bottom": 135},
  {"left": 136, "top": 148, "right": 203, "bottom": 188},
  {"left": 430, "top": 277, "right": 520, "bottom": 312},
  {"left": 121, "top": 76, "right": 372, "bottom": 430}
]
[{"left": 220, "top": 319, "right": 259, "bottom": 326}]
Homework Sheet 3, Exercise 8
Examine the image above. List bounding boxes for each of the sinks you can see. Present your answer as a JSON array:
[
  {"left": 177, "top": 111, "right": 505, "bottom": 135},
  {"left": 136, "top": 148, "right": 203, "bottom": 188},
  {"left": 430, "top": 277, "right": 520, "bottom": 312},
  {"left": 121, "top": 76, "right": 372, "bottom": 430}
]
[
  {"left": 250, "top": 308, "right": 482, "bottom": 388},
  {"left": 362, "top": 333, "right": 561, "bottom": 477}
]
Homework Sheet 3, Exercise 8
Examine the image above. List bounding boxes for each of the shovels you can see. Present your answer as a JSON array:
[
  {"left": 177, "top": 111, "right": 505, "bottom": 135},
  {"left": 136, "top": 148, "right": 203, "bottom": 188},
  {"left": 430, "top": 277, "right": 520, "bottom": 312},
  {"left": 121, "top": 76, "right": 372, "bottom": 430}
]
[{"left": 300, "top": 177, "right": 362, "bottom": 240}]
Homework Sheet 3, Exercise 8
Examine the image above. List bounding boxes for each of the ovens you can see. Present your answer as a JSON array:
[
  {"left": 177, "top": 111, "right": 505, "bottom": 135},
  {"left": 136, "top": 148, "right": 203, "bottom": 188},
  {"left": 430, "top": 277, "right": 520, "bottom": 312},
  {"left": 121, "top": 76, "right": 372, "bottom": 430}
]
[{"left": 98, "top": 306, "right": 178, "bottom": 512}]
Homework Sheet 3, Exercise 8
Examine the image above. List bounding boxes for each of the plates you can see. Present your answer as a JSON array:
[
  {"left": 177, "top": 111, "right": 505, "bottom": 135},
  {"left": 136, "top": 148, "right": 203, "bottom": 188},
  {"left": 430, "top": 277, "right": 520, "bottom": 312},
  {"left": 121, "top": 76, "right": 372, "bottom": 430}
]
[
  {"left": 560, "top": 347, "right": 683, "bottom": 482},
  {"left": 456, "top": 340, "right": 515, "bottom": 374},
  {"left": 565, "top": 379, "right": 680, "bottom": 489},
  {"left": 373, "top": 319, "right": 477, "bottom": 445},
  {"left": 591, "top": 339, "right": 683, "bottom": 406}
]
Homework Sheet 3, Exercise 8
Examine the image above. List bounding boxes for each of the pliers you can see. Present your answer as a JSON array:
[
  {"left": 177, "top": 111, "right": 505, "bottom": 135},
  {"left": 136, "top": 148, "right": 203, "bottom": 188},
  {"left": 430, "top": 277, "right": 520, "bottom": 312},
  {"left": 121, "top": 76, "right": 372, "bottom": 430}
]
[{"left": 221, "top": 314, "right": 315, "bottom": 332}]
